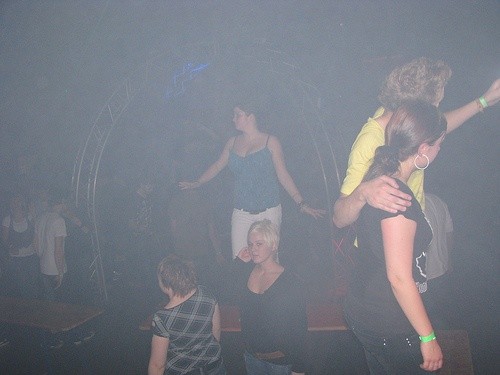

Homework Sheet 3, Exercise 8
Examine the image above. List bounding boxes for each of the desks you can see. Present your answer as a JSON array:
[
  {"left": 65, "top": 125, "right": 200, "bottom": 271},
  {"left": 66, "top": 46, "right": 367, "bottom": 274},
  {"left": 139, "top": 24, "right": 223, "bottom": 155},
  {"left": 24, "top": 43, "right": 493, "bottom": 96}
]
[{"left": 0, "top": 298, "right": 104, "bottom": 375}]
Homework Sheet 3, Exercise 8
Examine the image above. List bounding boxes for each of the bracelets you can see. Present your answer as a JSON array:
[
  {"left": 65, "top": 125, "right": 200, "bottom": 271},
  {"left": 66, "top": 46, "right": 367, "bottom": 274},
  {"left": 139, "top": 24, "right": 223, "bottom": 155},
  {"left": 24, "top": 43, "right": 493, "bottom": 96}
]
[
  {"left": 419, "top": 332, "right": 437, "bottom": 342},
  {"left": 475, "top": 95, "right": 489, "bottom": 111},
  {"left": 291, "top": 193, "right": 308, "bottom": 211}
]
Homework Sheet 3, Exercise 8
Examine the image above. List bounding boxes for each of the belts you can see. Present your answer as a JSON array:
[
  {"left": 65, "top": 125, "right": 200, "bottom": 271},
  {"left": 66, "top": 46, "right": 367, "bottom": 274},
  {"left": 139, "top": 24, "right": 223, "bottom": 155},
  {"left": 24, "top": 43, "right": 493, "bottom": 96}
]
[{"left": 234, "top": 205, "right": 275, "bottom": 215}]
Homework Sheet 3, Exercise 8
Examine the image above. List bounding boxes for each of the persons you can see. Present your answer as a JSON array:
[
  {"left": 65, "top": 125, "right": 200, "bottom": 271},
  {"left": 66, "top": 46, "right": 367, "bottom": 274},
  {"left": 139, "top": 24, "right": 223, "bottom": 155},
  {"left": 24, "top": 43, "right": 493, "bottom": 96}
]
[
  {"left": 225, "top": 219, "right": 310, "bottom": 375},
  {"left": 1, "top": 176, "right": 154, "bottom": 348},
  {"left": 341, "top": 100, "right": 448, "bottom": 375},
  {"left": 331, "top": 57, "right": 500, "bottom": 249},
  {"left": 177, "top": 102, "right": 327, "bottom": 264},
  {"left": 145, "top": 254, "right": 226, "bottom": 374}
]
